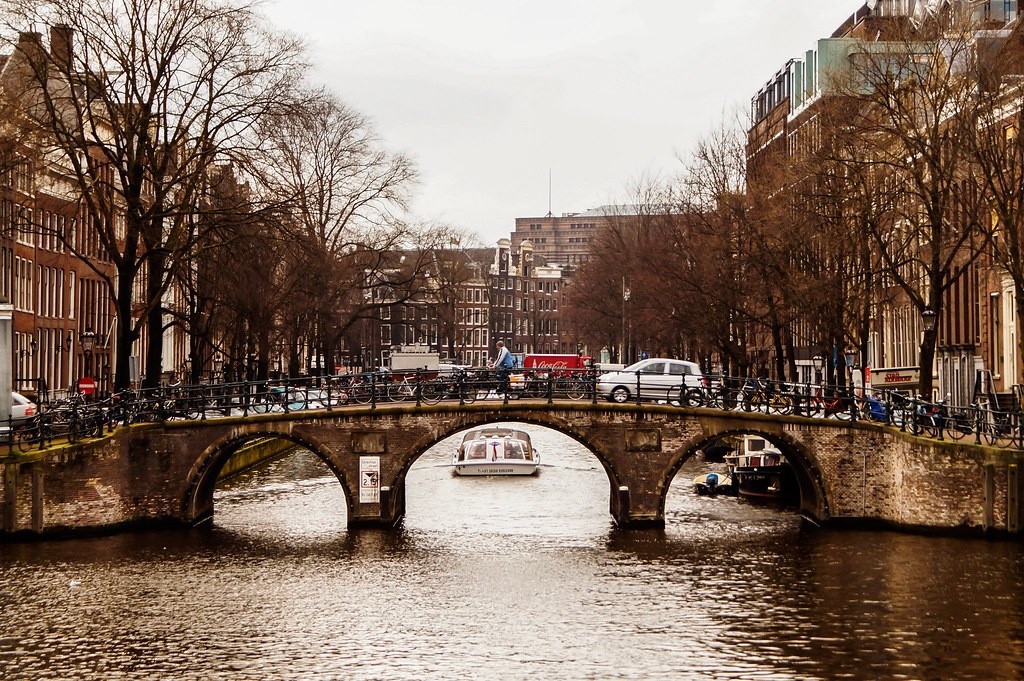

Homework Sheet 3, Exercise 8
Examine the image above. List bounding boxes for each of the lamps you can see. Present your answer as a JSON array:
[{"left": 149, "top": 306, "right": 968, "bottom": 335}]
[
  {"left": 20, "top": 337, "right": 38, "bottom": 358},
  {"left": 56, "top": 334, "right": 72, "bottom": 352},
  {"left": 921, "top": 305, "right": 936, "bottom": 337}
]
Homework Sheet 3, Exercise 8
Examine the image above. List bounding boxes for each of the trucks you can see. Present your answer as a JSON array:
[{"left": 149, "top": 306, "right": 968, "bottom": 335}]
[{"left": 524, "top": 354, "right": 596, "bottom": 377}]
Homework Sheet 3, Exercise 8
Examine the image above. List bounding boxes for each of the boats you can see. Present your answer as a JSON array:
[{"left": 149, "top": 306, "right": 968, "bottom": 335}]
[
  {"left": 691, "top": 473, "right": 732, "bottom": 494},
  {"left": 724, "top": 433, "right": 791, "bottom": 505},
  {"left": 452, "top": 427, "right": 540, "bottom": 476}
]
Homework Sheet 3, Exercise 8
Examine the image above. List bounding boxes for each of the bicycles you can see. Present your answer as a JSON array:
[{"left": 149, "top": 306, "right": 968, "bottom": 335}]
[
  {"left": 17, "top": 378, "right": 307, "bottom": 451},
  {"left": 318, "top": 365, "right": 606, "bottom": 401},
  {"left": 667, "top": 373, "right": 1023, "bottom": 449}
]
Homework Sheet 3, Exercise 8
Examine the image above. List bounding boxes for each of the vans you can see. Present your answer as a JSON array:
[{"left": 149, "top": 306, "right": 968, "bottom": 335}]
[
  {"left": 365, "top": 366, "right": 390, "bottom": 379},
  {"left": 437, "top": 365, "right": 474, "bottom": 378}
]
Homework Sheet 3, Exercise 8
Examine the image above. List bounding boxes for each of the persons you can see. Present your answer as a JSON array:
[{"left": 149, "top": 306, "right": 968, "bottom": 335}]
[{"left": 496, "top": 340, "right": 513, "bottom": 395}]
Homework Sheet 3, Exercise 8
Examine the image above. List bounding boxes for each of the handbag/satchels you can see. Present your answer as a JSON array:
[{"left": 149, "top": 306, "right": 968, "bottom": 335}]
[{"left": 510, "top": 375, "right": 525, "bottom": 390}]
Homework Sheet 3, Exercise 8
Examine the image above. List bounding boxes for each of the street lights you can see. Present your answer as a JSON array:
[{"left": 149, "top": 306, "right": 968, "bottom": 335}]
[
  {"left": 812, "top": 354, "right": 824, "bottom": 386},
  {"left": 845, "top": 348, "right": 857, "bottom": 419},
  {"left": 81, "top": 328, "right": 94, "bottom": 406},
  {"left": 921, "top": 308, "right": 938, "bottom": 403}
]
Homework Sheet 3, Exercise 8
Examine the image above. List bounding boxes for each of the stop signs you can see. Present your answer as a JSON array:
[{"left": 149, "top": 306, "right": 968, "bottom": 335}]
[{"left": 80, "top": 377, "right": 95, "bottom": 395}]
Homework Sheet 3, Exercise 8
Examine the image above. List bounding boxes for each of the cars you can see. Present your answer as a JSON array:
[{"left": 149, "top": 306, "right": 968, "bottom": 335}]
[
  {"left": 150, "top": 385, "right": 339, "bottom": 422},
  {"left": 11, "top": 392, "right": 38, "bottom": 425},
  {"left": 593, "top": 357, "right": 708, "bottom": 404}
]
